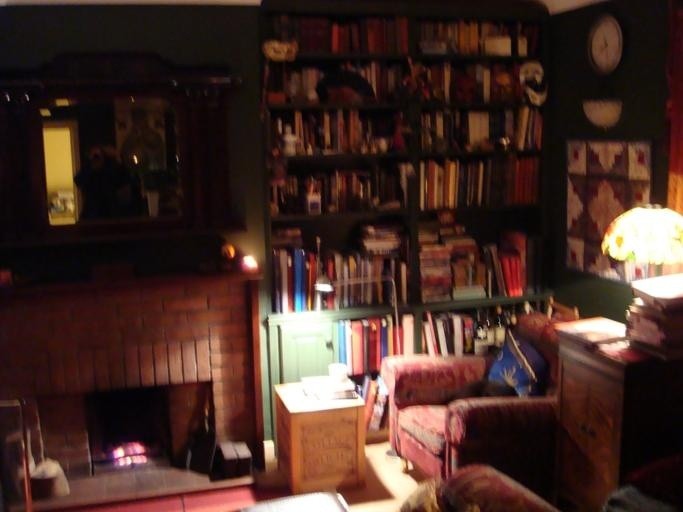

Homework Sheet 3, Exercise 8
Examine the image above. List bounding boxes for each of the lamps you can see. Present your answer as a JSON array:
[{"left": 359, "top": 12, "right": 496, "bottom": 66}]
[
  {"left": 598, "top": 205, "right": 683, "bottom": 267},
  {"left": 315, "top": 275, "right": 401, "bottom": 356}
]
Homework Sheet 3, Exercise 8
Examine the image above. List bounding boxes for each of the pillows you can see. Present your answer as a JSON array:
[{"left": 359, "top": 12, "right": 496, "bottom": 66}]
[{"left": 488, "top": 326, "right": 546, "bottom": 397}]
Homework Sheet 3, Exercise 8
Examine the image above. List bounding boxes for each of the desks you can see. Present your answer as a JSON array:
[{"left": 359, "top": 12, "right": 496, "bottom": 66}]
[{"left": 555, "top": 315, "right": 668, "bottom": 486}]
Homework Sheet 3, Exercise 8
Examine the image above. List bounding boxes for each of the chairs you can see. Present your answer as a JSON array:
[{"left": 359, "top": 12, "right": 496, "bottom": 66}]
[
  {"left": 400, "top": 463, "right": 561, "bottom": 512},
  {"left": 380, "top": 312, "right": 564, "bottom": 486}
]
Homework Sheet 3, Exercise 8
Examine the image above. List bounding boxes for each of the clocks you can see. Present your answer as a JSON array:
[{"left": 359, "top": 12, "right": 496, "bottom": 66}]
[{"left": 586, "top": 15, "right": 623, "bottom": 76}]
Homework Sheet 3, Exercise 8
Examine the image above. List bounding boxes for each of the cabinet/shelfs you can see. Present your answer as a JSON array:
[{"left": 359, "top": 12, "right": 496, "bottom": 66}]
[
  {"left": 411, "top": 0, "right": 550, "bottom": 311},
  {"left": 259, "top": 1, "right": 411, "bottom": 317}
]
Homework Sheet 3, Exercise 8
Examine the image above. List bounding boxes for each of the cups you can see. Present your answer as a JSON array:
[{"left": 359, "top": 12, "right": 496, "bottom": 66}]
[{"left": 328, "top": 364, "right": 347, "bottom": 382}]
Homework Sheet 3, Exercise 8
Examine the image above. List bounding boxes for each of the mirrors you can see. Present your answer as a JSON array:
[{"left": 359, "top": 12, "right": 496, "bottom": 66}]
[{"left": 0, "top": 50, "right": 245, "bottom": 249}]
